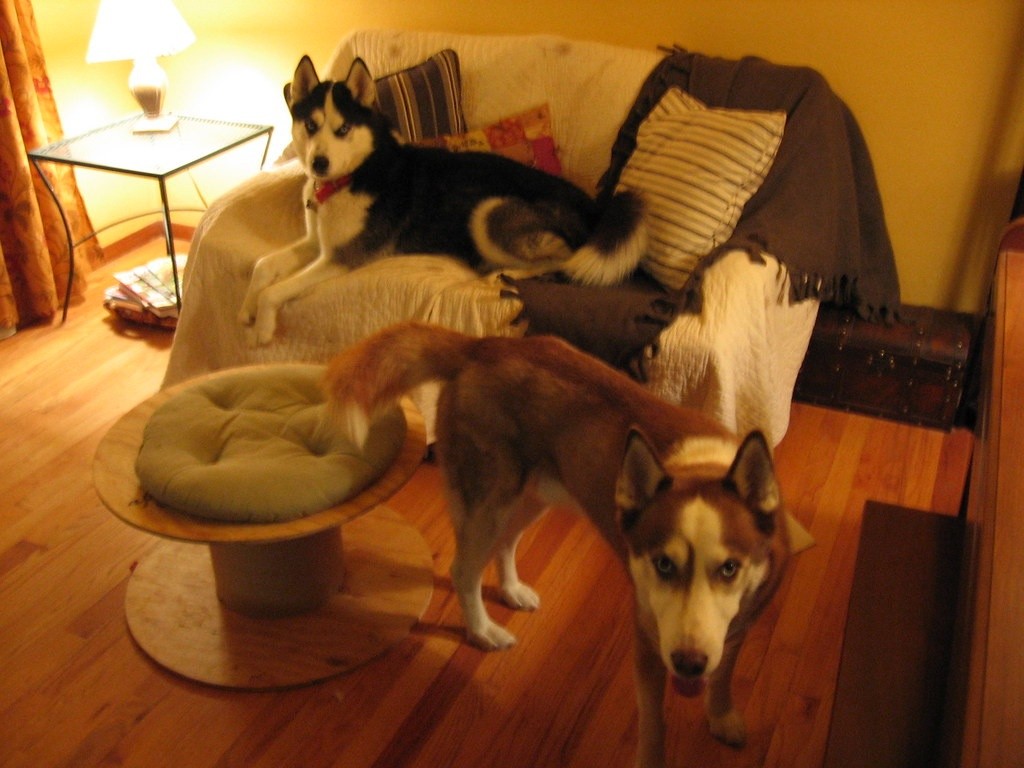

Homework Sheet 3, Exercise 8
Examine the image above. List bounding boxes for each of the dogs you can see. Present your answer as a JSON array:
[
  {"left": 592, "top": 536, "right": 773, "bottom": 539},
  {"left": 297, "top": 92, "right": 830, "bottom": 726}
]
[
  {"left": 331, "top": 322, "right": 791, "bottom": 768},
  {"left": 238, "top": 54, "right": 650, "bottom": 347}
]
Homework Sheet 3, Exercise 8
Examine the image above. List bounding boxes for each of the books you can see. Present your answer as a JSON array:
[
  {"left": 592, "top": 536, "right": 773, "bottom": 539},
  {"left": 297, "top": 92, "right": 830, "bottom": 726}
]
[
  {"left": 103, "top": 265, "right": 178, "bottom": 328},
  {"left": 146, "top": 256, "right": 188, "bottom": 300}
]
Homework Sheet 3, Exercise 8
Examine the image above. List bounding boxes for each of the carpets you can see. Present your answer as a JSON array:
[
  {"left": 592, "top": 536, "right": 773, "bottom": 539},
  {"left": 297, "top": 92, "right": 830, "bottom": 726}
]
[{"left": 824, "top": 499, "right": 961, "bottom": 768}]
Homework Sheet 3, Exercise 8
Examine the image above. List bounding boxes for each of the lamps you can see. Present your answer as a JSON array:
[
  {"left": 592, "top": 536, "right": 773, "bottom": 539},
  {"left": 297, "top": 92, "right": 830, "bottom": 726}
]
[{"left": 86, "top": 1, "right": 196, "bottom": 133}]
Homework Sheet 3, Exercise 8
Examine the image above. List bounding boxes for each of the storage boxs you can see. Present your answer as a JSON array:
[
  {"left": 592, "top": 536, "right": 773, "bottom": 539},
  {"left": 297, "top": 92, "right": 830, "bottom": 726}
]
[{"left": 791, "top": 303, "right": 977, "bottom": 432}]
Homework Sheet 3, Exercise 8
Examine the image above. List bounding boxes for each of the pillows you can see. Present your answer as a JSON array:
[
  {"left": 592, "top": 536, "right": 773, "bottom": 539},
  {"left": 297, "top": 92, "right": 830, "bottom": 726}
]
[
  {"left": 372, "top": 49, "right": 467, "bottom": 143},
  {"left": 158, "top": 31, "right": 915, "bottom": 458},
  {"left": 406, "top": 104, "right": 567, "bottom": 179},
  {"left": 616, "top": 87, "right": 785, "bottom": 294},
  {"left": 136, "top": 364, "right": 409, "bottom": 523}
]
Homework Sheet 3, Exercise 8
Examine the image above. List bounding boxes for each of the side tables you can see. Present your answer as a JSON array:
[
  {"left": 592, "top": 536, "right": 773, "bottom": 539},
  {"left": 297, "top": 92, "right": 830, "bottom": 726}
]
[{"left": 27, "top": 110, "right": 274, "bottom": 322}]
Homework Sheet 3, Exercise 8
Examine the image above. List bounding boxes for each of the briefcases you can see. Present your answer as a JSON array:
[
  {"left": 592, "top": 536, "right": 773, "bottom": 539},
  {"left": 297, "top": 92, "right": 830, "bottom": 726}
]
[{"left": 793, "top": 299, "right": 976, "bottom": 433}]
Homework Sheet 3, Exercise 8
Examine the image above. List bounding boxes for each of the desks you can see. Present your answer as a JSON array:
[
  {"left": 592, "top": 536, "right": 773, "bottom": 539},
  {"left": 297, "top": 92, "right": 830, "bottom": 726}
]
[
  {"left": 93, "top": 368, "right": 431, "bottom": 690},
  {"left": 963, "top": 220, "right": 1024, "bottom": 768}
]
